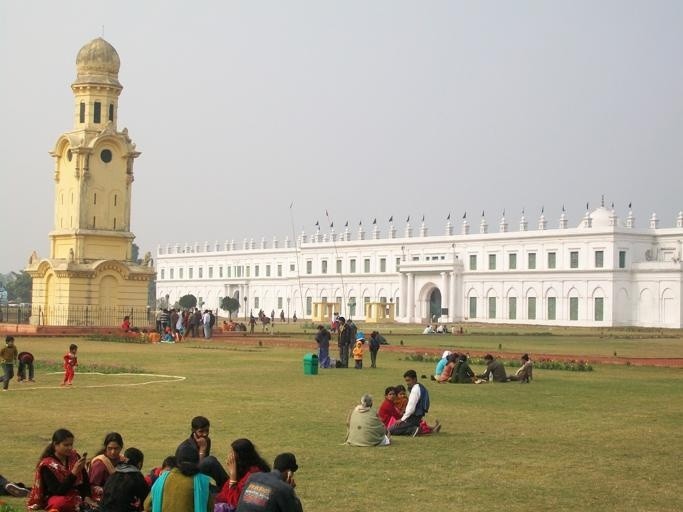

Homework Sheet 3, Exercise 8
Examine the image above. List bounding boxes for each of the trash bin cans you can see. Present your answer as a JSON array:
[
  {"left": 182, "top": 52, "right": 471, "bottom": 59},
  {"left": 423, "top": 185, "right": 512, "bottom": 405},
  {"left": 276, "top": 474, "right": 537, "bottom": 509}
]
[{"left": 304, "top": 353, "right": 318, "bottom": 375}]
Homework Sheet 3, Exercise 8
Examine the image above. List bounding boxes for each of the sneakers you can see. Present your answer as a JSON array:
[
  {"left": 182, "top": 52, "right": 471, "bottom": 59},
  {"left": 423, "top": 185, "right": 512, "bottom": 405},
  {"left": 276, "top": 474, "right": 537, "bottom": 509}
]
[{"left": 433, "top": 420, "right": 441, "bottom": 433}]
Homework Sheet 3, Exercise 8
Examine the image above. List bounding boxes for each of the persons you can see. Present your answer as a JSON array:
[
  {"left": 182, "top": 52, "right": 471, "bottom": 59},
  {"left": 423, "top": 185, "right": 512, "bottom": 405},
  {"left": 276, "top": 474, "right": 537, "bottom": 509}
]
[
  {"left": 507, "top": 353, "right": 532, "bottom": 382},
  {"left": 423, "top": 324, "right": 464, "bottom": 336},
  {"left": 1, "top": 335, "right": 18, "bottom": 390},
  {"left": 476, "top": 354, "right": 506, "bottom": 382},
  {"left": 315, "top": 317, "right": 386, "bottom": 369},
  {"left": 221, "top": 309, "right": 285, "bottom": 331},
  {"left": 378, "top": 370, "right": 441, "bottom": 437},
  {"left": 434, "top": 350, "right": 474, "bottom": 384},
  {"left": 61, "top": 344, "right": 78, "bottom": 386},
  {"left": 344, "top": 393, "right": 390, "bottom": 447},
  {"left": 293, "top": 313, "right": 297, "bottom": 322},
  {"left": 122, "top": 308, "right": 215, "bottom": 343},
  {"left": 17, "top": 352, "right": 35, "bottom": 382},
  {"left": 1, "top": 416, "right": 303, "bottom": 511}
]
